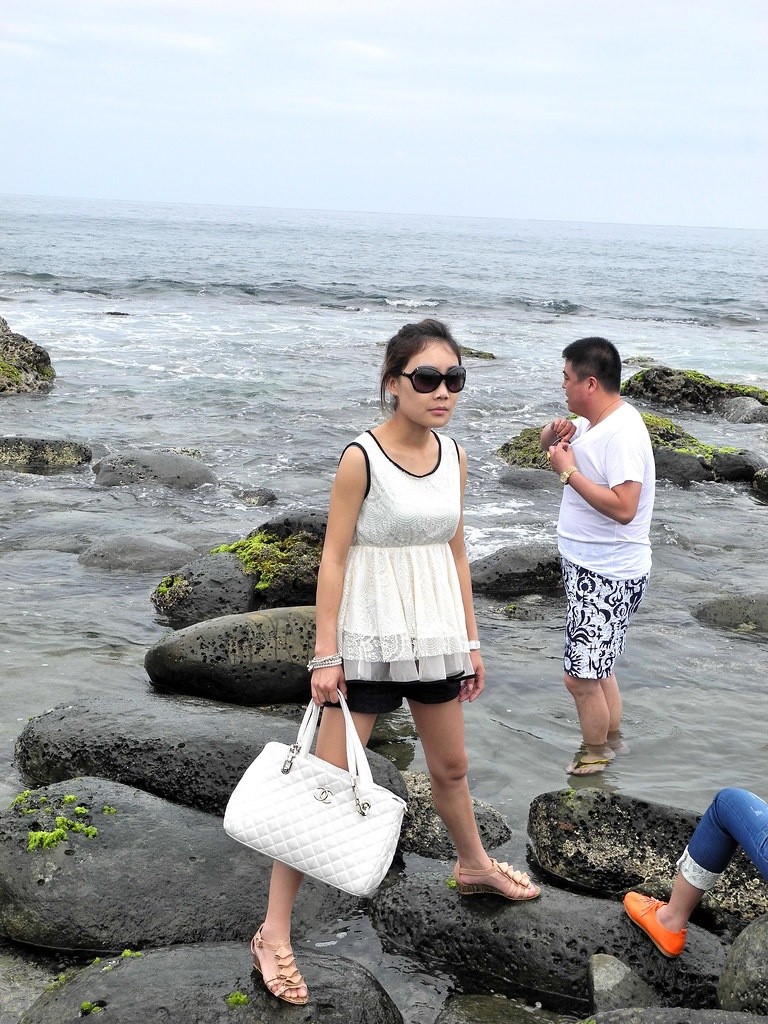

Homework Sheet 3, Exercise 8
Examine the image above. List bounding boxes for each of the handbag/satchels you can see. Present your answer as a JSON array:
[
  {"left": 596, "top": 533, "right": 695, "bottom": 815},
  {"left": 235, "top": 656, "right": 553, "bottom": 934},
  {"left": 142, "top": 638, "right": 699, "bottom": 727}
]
[{"left": 221, "top": 682, "right": 407, "bottom": 899}]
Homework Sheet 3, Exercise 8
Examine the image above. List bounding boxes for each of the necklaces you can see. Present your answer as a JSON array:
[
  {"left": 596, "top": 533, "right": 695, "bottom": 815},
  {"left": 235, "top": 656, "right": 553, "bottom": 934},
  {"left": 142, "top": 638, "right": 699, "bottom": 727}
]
[{"left": 586, "top": 399, "right": 622, "bottom": 432}]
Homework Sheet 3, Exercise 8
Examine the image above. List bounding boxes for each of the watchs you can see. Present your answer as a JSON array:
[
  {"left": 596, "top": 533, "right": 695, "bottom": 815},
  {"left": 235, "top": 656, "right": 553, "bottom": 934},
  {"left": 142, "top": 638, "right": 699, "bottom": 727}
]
[{"left": 560, "top": 466, "right": 579, "bottom": 485}]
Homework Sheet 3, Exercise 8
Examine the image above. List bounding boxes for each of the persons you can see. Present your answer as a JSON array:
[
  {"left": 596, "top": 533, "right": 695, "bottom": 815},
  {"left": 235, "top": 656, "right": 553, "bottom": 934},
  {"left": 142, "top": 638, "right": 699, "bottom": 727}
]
[
  {"left": 250, "top": 319, "right": 542, "bottom": 1006},
  {"left": 624, "top": 787, "right": 768, "bottom": 959},
  {"left": 540, "top": 337, "right": 656, "bottom": 777}
]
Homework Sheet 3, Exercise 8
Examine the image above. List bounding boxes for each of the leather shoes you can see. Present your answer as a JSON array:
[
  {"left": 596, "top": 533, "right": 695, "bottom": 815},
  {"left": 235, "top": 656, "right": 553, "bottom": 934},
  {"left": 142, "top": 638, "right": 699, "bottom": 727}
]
[{"left": 624, "top": 890, "right": 688, "bottom": 959}]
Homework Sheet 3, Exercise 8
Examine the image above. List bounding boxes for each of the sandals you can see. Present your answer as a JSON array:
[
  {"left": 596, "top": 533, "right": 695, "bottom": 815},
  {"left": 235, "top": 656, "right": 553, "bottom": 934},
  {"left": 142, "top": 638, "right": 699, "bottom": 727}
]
[
  {"left": 452, "top": 855, "right": 542, "bottom": 900},
  {"left": 250, "top": 923, "right": 308, "bottom": 1006}
]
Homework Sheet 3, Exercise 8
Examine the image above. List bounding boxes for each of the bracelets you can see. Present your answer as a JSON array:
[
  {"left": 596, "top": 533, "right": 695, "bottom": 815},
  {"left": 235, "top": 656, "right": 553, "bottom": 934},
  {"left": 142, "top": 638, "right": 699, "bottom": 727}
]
[
  {"left": 306, "top": 652, "right": 343, "bottom": 672},
  {"left": 470, "top": 640, "right": 481, "bottom": 651}
]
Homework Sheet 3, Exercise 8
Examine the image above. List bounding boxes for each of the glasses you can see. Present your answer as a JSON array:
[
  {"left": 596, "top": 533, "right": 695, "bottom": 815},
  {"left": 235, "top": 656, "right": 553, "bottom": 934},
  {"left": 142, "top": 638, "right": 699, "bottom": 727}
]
[{"left": 400, "top": 366, "right": 465, "bottom": 393}]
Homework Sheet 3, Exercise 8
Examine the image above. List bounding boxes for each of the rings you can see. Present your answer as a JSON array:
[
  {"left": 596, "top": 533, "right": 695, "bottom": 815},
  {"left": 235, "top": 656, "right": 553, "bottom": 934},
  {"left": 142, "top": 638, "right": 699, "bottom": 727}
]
[{"left": 547, "top": 453, "right": 550, "bottom": 459}]
